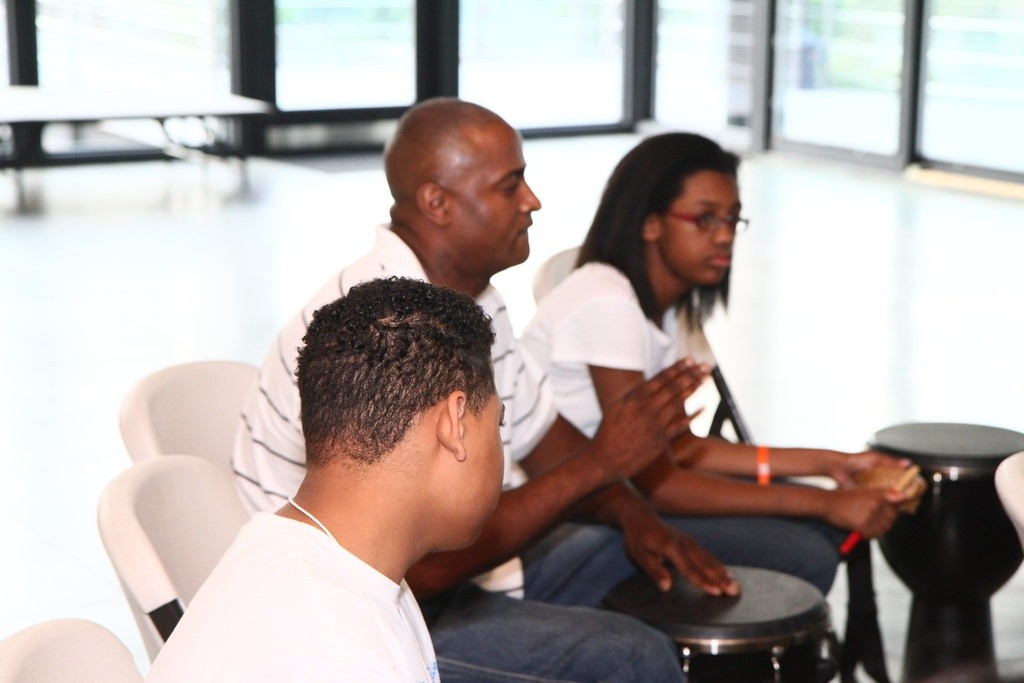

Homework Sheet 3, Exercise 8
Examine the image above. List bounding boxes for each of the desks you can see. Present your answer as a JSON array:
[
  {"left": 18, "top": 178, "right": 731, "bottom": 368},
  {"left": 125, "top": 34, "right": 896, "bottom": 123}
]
[{"left": 0, "top": 86, "right": 278, "bottom": 214}]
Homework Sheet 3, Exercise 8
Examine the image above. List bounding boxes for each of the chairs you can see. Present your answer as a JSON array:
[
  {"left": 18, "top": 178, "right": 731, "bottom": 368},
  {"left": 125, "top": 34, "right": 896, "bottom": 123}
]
[
  {"left": 534, "top": 247, "right": 896, "bottom": 683},
  {"left": 99, "top": 454, "right": 250, "bottom": 667},
  {"left": 0, "top": 618, "right": 143, "bottom": 683},
  {"left": 119, "top": 360, "right": 258, "bottom": 480}
]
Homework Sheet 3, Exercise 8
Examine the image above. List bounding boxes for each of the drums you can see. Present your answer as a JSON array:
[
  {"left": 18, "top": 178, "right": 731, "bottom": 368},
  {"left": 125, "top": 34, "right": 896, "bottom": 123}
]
[
  {"left": 870, "top": 423, "right": 1024, "bottom": 682},
  {"left": 608, "top": 562, "right": 834, "bottom": 682}
]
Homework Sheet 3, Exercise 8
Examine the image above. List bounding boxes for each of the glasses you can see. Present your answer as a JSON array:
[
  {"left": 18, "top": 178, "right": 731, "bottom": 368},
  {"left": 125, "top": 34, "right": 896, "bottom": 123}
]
[{"left": 668, "top": 210, "right": 748, "bottom": 234}]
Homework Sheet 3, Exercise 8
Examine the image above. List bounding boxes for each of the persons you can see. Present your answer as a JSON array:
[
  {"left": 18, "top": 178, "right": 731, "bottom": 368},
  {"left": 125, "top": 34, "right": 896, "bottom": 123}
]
[
  {"left": 517, "top": 132, "right": 925, "bottom": 593},
  {"left": 231, "top": 98, "right": 741, "bottom": 683},
  {"left": 143, "top": 276, "right": 505, "bottom": 683}
]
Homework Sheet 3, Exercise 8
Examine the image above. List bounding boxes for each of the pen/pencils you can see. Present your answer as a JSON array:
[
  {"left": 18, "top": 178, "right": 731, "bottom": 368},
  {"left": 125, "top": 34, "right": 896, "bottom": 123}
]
[
  {"left": 756, "top": 448, "right": 770, "bottom": 485},
  {"left": 840, "top": 465, "right": 919, "bottom": 554}
]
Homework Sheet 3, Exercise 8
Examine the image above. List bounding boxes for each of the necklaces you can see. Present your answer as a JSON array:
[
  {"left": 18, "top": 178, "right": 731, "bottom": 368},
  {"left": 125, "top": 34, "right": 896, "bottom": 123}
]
[{"left": 288, "top": 496, "right": 338, "bottom": 546}]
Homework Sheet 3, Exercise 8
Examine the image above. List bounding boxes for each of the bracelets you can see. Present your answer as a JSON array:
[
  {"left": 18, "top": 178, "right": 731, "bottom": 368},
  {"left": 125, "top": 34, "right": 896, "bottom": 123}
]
[{"left": 756, "top": 446, "right": 770, "bottom": 487}]
